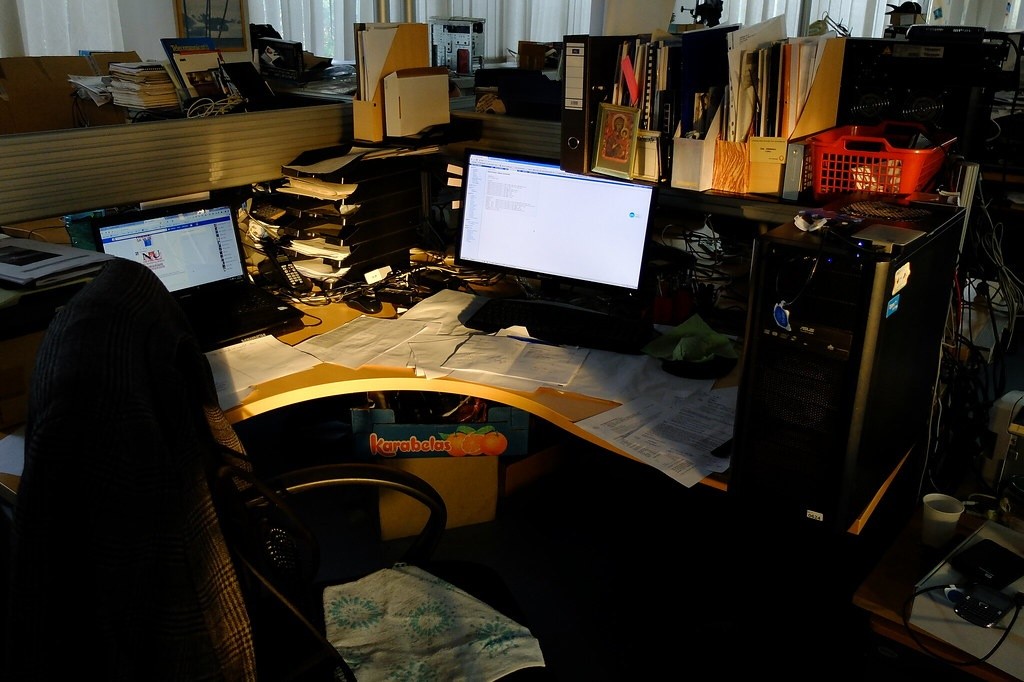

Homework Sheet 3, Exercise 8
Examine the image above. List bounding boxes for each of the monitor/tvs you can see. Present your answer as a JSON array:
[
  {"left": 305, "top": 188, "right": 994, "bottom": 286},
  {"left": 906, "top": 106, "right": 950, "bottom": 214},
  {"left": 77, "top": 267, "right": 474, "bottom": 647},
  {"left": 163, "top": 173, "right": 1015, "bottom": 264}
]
[{"left": 456, "top": 146, "right": 662, "bottom": 315}]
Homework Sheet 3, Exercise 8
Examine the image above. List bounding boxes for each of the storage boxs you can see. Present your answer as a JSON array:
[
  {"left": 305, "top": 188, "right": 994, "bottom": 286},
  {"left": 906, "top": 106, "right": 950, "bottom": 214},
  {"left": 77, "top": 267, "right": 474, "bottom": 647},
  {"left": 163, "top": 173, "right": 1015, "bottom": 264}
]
[
  {"left": 346, "top": 402, "right": 528, "bottom": 461},
  {"left": 351, "top": 22, "right": 431, "bottom": 143},
  {"left": 373, "top": 456, "right": 497, "bottom": 540},
  {"left": 712, "top": 108, "right": 749, "bottom": 196},
  {"left": 807, "top": 120, "right": 957, "bottom": 203},
  {"left": 667, "top": 95, "right": 724, "bottom": 192},
  {"left": 746, "top": 36, "right": 847, "bottom": 196}
]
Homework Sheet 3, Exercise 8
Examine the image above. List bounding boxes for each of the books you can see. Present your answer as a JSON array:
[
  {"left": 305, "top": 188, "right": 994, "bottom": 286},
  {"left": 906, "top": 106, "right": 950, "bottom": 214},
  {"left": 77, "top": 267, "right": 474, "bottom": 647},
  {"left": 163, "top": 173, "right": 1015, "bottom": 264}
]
[
  {"left": 109, "top": 61, "right": 179, "bottom": 109},
  {"left": 612, "top": 13, "right": 846, "bottom": 142}
]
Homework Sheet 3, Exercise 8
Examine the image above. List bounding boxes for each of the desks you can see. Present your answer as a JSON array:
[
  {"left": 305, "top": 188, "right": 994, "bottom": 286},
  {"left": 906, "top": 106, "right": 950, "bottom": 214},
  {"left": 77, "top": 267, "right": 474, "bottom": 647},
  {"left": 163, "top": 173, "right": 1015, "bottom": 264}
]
[{"left": 0, "top": 169, "right": 1024, "bottom": 682}]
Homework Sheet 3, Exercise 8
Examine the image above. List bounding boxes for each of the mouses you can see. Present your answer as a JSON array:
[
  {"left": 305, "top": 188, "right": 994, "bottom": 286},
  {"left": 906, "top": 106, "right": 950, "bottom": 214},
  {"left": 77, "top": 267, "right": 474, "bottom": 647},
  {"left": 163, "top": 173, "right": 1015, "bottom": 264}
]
[{"left": 344, "top": 291, "right": 383, "bottom": 314}]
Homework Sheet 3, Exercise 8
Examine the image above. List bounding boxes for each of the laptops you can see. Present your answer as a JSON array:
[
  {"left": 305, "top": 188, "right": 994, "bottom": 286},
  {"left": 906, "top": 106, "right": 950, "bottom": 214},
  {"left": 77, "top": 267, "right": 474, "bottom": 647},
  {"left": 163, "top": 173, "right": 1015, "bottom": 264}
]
[{"left": 92, "top": 197, "right": 305, "bottom": 353}]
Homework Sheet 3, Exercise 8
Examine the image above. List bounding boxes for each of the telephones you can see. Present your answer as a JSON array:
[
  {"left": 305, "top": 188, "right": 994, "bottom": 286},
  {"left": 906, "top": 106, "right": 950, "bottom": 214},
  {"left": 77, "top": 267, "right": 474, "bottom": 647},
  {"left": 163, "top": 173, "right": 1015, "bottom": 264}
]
[{"left": 261, "top": 236, "right": 309, "bottom": 294}]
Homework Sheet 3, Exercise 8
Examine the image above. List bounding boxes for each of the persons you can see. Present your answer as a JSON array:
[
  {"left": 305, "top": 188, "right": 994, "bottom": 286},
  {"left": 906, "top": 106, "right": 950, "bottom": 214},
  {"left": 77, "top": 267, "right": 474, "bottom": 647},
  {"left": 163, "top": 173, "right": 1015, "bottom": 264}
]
[{"left": 604, "top": 117, "right": 629, "bottom": 159}]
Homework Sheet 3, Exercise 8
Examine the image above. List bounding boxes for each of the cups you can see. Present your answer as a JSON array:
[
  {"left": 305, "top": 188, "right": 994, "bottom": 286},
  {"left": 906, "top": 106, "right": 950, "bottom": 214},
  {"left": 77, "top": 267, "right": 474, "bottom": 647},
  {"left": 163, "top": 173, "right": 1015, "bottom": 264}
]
[{"left": 920, "top": 493, "right": 965, "bottom": 547}]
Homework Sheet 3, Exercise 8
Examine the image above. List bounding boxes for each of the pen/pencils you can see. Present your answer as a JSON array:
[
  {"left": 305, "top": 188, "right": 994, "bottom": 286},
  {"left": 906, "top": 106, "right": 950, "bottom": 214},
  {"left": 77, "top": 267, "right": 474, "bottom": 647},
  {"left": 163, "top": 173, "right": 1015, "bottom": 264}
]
[{"left": 506, "top": 335, "right": 566, "bottom": 347}]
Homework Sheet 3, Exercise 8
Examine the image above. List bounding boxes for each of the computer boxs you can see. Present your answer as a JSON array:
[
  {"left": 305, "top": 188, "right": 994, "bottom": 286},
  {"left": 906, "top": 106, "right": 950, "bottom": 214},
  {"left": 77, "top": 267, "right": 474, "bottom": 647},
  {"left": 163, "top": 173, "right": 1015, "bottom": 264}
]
[
  {"left": 733, "top": 194, "right": 967, "bottom": 534},
  {"left": 842, "top": 26, "right": 1010, "bottom": 133}
]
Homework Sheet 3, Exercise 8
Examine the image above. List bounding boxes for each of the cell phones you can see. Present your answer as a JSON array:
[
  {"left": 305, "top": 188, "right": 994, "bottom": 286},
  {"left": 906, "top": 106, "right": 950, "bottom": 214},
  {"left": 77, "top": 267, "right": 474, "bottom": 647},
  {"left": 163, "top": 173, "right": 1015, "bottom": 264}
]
[{"left": 956, "top": 584, "right": 1016, "bottom": 629}]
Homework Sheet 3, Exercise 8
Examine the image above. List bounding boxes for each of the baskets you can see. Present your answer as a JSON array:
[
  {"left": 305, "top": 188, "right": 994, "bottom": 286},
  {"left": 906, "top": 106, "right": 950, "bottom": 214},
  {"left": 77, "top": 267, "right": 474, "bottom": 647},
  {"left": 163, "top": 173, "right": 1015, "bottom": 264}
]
[{"left": 806, "top": 124, "right": 957, "bottom": 203}]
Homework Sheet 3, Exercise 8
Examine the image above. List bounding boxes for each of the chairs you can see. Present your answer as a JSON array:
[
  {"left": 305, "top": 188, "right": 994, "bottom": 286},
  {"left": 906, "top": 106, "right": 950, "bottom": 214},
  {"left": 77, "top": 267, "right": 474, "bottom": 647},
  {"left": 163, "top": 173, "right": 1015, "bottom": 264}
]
[{"left": 0, "top": 258, "right": 551, "bottom": 682}]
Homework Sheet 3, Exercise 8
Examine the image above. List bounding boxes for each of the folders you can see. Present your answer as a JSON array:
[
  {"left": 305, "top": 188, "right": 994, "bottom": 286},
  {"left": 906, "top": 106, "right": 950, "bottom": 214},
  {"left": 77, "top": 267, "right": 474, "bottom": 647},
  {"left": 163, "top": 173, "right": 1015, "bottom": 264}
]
[{"left": 558, "top": 30, "right": 655, "bottom": 180}]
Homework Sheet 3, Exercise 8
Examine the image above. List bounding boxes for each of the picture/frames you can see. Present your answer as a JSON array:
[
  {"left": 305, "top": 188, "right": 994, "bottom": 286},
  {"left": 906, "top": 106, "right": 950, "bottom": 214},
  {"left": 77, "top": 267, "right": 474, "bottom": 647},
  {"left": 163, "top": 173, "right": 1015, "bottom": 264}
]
[
  {"left": 173, "top": 0, "right": 254, "bottom": 64},
  {"left": 592, "top": 101, "right": 641, "bottom": 179}
]
